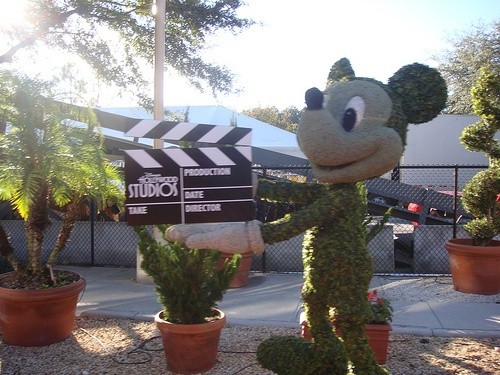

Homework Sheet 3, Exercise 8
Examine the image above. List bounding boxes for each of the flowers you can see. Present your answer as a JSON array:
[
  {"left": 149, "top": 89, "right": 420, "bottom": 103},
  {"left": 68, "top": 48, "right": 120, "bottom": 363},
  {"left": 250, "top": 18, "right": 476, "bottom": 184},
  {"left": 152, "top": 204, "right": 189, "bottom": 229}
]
[{"left": 365, "top": 290, "right": 393, "bottom": 325}]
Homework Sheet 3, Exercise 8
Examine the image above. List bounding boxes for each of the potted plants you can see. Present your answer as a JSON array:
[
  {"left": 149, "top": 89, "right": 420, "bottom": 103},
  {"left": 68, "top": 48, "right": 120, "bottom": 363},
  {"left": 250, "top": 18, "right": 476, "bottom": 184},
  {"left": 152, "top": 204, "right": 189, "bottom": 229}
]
[
  {"left": 0, "top": 67, "right": 126, "bottom": 346},
  {"left": 445, "top": 64, "right": 500, "bottom": 296},
  {"left": 130, "top": 223, "right": 242, "bottom": 375}
]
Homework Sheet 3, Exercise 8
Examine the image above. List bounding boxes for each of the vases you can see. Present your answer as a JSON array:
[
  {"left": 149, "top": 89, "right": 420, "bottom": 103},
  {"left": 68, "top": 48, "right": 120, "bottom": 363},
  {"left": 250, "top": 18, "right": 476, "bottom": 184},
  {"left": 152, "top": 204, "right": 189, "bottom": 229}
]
[{"left": 300, "top": 311, "right": 393, "bottom": 365}]
